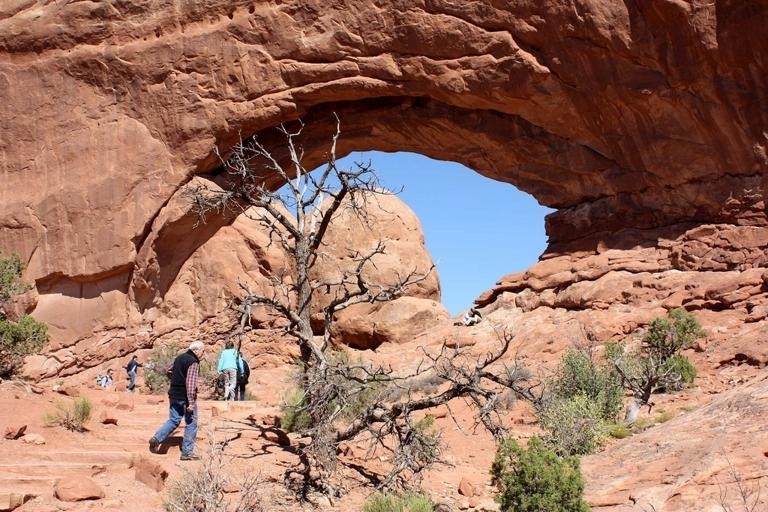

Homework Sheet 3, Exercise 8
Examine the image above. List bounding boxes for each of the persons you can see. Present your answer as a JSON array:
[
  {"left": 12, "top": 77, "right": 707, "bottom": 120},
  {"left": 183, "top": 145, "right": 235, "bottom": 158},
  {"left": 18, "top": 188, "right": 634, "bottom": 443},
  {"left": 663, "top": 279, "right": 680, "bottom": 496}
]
[
  {"left": 465, "top": 306, "right": 483, "bottom": 327},
  {"left": 148, "top": 340, "right": 204, "bottom": 459},
  {"left": 213, "top": 339, "right": 250, "bottom": 403},
  {"left": 94, "top": 369, "right": 115, "bottom": 390},
  {"left": 462, "top": 311, "right": 474, "bottom": 326},
  {"left": 126, "top": 355, "right": 146, "bottom": 392}
]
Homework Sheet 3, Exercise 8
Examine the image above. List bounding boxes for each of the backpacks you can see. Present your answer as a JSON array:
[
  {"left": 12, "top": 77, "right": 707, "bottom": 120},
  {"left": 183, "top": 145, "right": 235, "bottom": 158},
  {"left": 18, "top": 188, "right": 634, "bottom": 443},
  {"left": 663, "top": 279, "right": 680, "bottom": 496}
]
[{"left": 96, "top": 374, "right": 108, "bottom": 385}]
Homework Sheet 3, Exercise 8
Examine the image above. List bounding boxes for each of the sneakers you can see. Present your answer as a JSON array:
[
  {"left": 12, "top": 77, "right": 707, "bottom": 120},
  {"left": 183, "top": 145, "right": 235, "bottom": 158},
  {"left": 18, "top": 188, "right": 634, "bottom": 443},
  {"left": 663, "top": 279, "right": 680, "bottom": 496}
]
[
  {"left": 181, "top": 452, "right": 200, "bottom": 460},
  {"left": 149, "top": 436, "right": 158, "bottom": 453}
]
